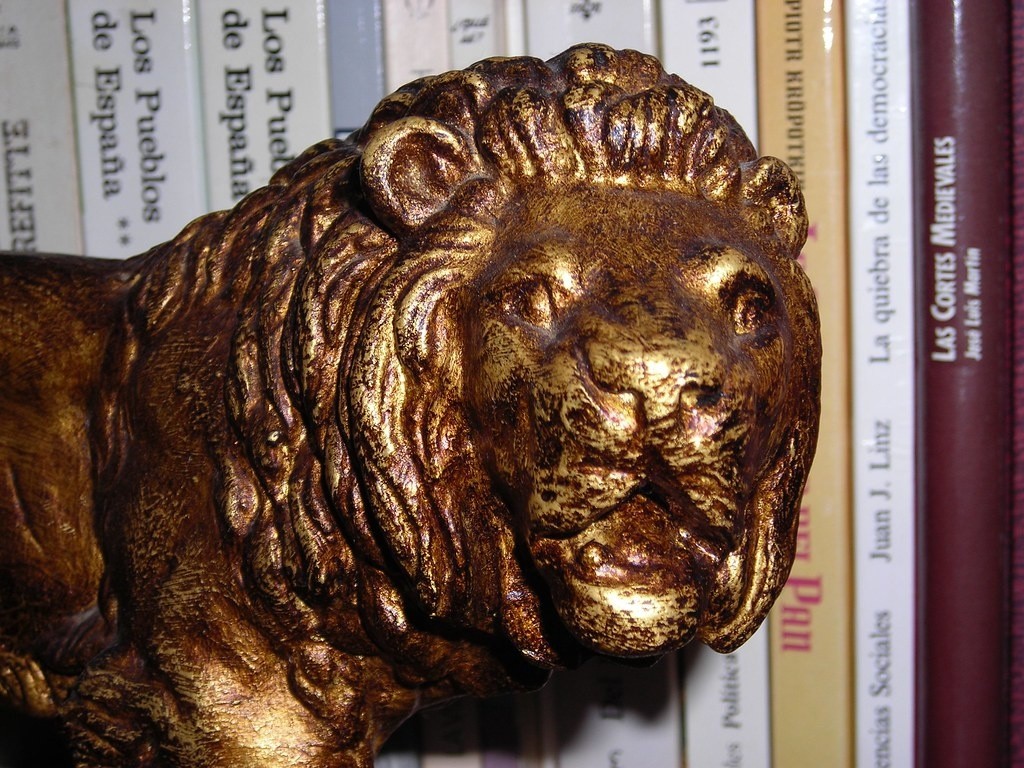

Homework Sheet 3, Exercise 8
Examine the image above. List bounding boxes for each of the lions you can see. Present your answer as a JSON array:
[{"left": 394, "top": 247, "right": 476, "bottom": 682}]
[{"left": 1, "top": 43, "right": 823, "bottom": 768}]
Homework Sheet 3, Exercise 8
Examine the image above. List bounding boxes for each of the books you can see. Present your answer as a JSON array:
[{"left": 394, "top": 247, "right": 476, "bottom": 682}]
[{"left": 1, "top": 0, "right": 1024, "bottom": 768}]
